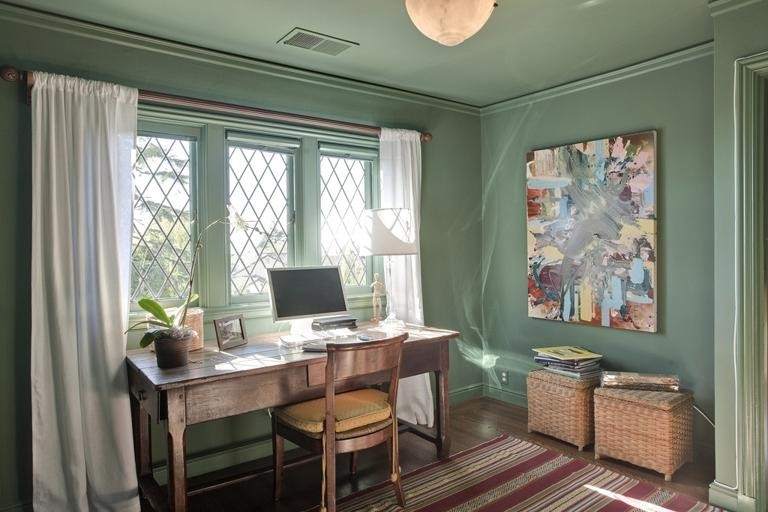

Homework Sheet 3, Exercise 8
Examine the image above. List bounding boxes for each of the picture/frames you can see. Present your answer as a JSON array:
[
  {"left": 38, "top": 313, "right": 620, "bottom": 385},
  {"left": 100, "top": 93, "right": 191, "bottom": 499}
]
[{"left": 213, "top": 314, "right": 248, "bottom": 351}]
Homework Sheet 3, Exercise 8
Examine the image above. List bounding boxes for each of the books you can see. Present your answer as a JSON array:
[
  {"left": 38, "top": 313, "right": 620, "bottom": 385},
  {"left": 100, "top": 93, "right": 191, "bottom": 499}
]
[{"left": 532, "top": 346, "right": 604, "bottom": 379}]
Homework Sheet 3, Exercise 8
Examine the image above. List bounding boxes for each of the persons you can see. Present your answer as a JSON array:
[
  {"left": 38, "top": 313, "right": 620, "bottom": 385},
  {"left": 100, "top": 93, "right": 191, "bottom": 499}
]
[{"left": 370, "top": 273, "right": 386, "bottom": 321}]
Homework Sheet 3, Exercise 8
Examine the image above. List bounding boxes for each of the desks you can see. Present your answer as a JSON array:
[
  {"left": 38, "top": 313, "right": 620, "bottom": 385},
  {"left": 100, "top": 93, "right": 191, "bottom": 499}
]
[{"left": 125, "top": 318, "right": 460, "bottom": 512}]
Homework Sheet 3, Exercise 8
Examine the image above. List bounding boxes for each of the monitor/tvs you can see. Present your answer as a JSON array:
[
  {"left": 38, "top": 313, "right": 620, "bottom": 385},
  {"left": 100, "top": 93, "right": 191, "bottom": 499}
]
[{"left": 267, "top": 265, "right": 350, "bottom": 337}]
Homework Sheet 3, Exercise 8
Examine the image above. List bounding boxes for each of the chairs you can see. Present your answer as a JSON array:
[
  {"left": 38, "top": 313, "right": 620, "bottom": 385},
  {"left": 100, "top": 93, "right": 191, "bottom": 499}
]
[{"left": 269, "top": 332, "right": 409, "bottom": 512}]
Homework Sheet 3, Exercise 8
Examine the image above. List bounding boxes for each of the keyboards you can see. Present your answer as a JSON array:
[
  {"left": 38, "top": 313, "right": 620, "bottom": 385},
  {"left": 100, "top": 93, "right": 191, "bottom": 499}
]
[{"left": 280, "top": 326, "right": 353, "bottom": 347}]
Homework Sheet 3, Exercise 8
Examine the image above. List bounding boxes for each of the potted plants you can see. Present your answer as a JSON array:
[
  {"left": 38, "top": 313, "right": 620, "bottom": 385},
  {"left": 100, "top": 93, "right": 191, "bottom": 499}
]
[{"left": 123, "top": 293, "right": 200, "bottom": 369}]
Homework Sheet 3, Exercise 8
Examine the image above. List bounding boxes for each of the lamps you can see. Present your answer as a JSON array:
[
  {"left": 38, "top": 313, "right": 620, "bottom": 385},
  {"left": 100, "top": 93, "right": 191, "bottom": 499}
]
[
  {"left": 406, "top": 0, "right": 496, "bottom": 48},
  {"left": 359, "top": 207, "right": 419, "bottom": 311}
]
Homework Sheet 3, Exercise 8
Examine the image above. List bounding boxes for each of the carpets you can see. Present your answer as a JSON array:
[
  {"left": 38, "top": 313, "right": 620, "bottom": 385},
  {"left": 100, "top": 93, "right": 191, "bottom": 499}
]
[{"left": 305, "top": 434, "right": 733, "bottom": 512}]
